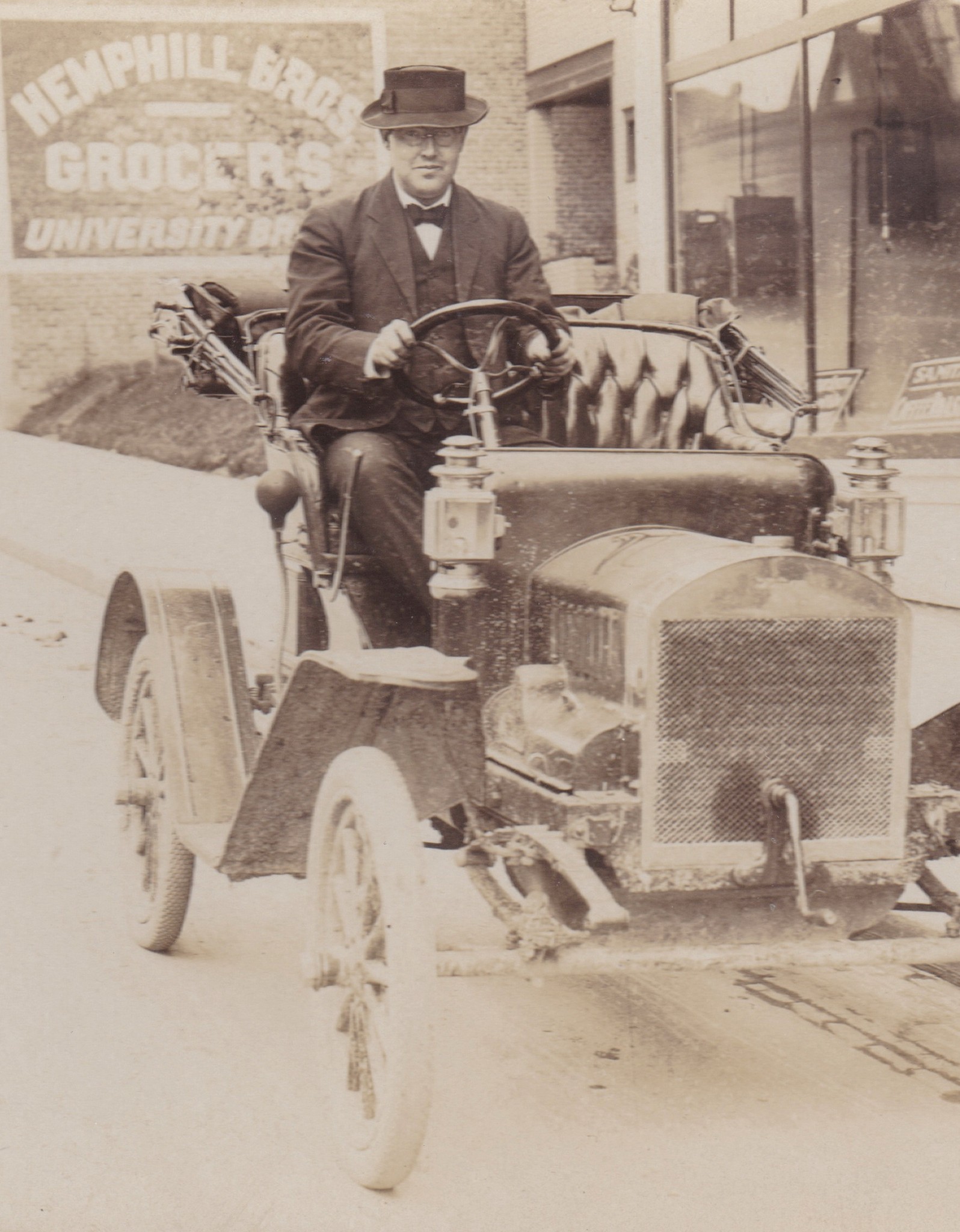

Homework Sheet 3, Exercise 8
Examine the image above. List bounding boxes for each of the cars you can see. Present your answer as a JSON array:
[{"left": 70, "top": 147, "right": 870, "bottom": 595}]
[{"left": 91, "top": 274, "right": 960, "bottom": 1192}]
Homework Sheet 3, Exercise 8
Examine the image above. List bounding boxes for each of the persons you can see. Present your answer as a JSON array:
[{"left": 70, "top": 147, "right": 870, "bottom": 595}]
[{"left": 282, "top": 65, "right": 581, "bottom": 620}]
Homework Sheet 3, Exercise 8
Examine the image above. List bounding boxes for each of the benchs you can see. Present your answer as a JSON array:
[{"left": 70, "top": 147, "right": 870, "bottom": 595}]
[{"left": 259, "top": 318, "right": 766, "bottom": 575}]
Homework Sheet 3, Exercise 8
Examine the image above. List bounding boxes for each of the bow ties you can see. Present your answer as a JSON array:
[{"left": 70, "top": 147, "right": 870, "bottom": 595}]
[{"left": 407, "top": 204, "right": 449, "bottom": 228}]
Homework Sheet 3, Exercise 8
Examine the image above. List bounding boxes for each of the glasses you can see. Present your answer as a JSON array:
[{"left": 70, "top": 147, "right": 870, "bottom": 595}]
[{"left": 391, "top": 126, "right": 462, "bottom": 147}]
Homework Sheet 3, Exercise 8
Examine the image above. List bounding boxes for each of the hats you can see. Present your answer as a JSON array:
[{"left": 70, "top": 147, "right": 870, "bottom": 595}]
[{"left": 359, "top": 64, "right": 490, "bottom": 130}]
